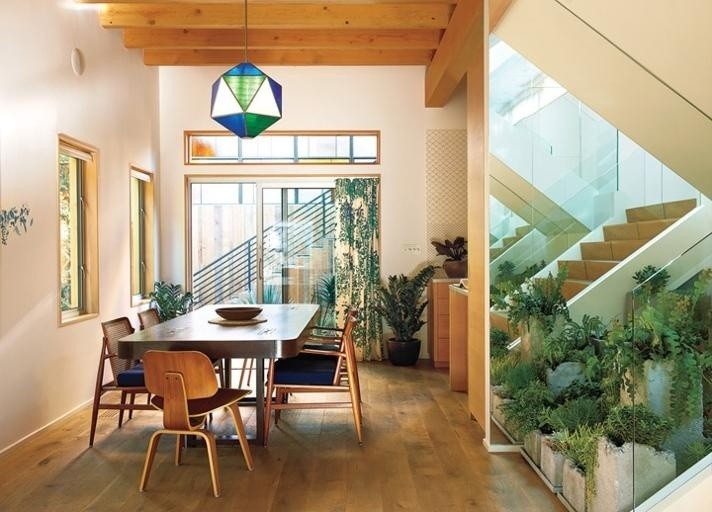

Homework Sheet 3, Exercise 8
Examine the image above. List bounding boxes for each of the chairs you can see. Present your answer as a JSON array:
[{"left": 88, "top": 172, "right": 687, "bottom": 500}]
[
  {"left": 274, "top": 309, "right": 362, "bottom": 418},
  {"left": 263, "top": 315, "right": 363, "bottom": 448},
  {"left": 138, "top": 309, "right": 225, "bottom": 390},
  {"left": 89, "top": 316, "right": 158, "bottom": 446},
  {"left": 139, "top": 350, "right": 255, "bottom": 498}
]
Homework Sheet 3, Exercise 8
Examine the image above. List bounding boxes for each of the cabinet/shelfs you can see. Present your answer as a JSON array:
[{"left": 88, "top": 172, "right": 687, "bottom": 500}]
[
  {"left": 427, "top": 276, "right": 466, "bottom": 368},
  {"left": 449, "top": 282, "right": 468, "bottom": 393}
]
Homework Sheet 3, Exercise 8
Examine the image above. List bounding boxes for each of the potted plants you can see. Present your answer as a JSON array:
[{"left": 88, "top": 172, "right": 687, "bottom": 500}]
[
  {"left": 430, "top": 237, "right": 468, "bottom": 277},
  {"left": 372, "top": 265, "right": 442, "bottom": 367}
]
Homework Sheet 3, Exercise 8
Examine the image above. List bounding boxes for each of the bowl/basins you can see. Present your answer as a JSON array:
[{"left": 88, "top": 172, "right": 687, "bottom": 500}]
[{"left": 215, "top": 307, "right": 262, "bottom": 320}]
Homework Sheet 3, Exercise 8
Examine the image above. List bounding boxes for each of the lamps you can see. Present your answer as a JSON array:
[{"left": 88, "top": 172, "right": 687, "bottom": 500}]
[{"left": 211, "top": 0, "right": 282, "bottom": 139}]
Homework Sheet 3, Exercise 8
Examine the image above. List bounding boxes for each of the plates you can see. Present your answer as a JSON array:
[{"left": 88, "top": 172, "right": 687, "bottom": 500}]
[{"left": 208, "top": 317, "right": 267, "bottom": 327}]
